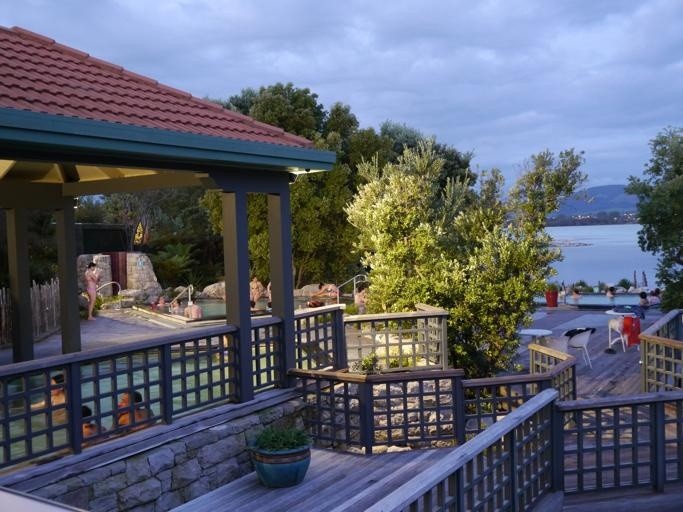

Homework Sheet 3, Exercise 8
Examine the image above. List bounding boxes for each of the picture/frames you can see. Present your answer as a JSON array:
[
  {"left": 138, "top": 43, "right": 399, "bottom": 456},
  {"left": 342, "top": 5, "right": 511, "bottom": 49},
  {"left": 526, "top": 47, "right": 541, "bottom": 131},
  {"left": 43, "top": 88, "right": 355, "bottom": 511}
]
[
  {"left": 515, "top": 328, "right": 552, "bottom": 345},
  {"left": 604, "top": 308, "right": 645, "bottom": 318}
]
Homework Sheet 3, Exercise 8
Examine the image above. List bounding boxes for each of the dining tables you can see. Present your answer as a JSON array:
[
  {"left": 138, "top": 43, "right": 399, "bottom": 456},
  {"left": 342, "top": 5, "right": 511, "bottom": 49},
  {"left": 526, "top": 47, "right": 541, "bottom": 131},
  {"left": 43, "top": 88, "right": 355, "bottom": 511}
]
[{"left": 248, "top": 421, "right": 312, "bottom": 488}]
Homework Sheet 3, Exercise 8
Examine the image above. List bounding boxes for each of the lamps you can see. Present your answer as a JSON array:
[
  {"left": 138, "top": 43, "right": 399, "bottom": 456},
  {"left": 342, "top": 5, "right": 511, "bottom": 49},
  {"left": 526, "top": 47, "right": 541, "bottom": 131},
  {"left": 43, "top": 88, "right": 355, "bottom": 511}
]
[
  {"left": 560, "top": 327, "right": 596, "bottom": 370},
  {"left": 608, "top": 317, "right": 640, "bottom": 352}
]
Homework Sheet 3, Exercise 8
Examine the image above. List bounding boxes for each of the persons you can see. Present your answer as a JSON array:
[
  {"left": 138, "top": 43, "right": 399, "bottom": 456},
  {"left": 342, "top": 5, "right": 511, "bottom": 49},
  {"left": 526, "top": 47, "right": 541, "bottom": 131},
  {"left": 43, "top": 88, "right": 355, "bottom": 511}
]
[
  {"left": 265, "top": 302, "right": 272, "bottom": 312},
  {"left": 156, "top": 296, "right": 170, "bottom": 306},
  {"left": 354, "top": 285, "right": 366, "bottom": 314},
  {"left": 184, "top": 296, "right": 202, "bottom": 319},
  {"left": 29, "top": 373, "right": 64, "bottom": 417},
  {"left": 606, "top": 286, "right": 615, "bottom": 297},
  {"left": 168, "top": 299, "right": 182, "bottom": 313},
  {"left": 648, "top": 290, "right": 660, "bottom": 304},
  {"left": 571, "top": 288, "right": 581, "bottom": 298},
  {"left": 638, "top": 290, "right": 649, "bottom": 305},
  {"left": 654, "top": 287, "right": 661, "bottom": 298},
  {"left": 266, "top": 281, "right": 272, "bottom": 301},
  {"left": 117, "top": 390, "right": 155, "bottom": 430},
  {"left": 310, "top": 282, "right": 342, "bottom": 298},
  {"left": 249, "top": 300, "right": 258, "bottom": 310},
  {"left": 63, "top": 405, "right": 109, "bottom": 452},
  {"left": 84, "top": 262, "right": 100, "bottom": 321},
  {"left": 249, "top": 274, "right": 263, "bottom": 303}
]
